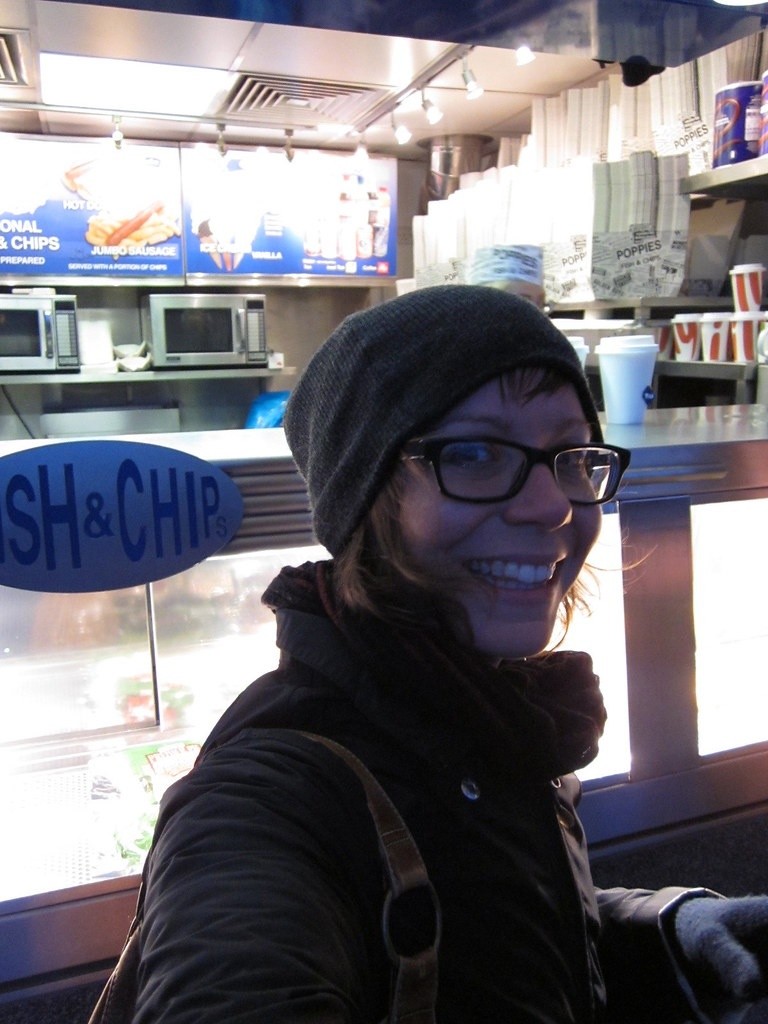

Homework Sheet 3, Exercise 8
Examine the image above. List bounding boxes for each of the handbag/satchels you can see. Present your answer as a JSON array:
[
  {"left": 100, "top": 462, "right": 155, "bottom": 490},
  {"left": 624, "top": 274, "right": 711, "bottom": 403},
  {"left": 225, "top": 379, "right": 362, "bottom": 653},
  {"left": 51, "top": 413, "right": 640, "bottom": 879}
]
[{"left": 69, "top": 726, "right": 443, "bottom": 1020}]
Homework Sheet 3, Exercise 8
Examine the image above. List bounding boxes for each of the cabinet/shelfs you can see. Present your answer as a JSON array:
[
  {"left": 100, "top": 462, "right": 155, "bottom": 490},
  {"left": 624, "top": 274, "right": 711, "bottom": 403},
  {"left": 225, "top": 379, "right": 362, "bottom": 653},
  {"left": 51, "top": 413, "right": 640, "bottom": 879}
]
[{"left": 644, "top": 151, "right": 768, "bottom": 407}]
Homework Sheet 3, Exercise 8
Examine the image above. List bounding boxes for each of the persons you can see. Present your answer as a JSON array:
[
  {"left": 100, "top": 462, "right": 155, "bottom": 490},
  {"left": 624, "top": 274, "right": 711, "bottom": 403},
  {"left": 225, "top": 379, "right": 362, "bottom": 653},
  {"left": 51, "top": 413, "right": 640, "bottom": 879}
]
[{"left": 133, "top": 288, "right": 768, "bottom": 1024}]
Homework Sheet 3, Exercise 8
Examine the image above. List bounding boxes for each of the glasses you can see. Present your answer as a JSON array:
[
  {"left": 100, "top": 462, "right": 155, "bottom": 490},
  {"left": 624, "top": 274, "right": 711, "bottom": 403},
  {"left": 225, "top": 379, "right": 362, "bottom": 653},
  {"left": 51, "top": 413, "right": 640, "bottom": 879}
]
[{"left": 393, "top": 428, "right": 633, "bottom": 509}]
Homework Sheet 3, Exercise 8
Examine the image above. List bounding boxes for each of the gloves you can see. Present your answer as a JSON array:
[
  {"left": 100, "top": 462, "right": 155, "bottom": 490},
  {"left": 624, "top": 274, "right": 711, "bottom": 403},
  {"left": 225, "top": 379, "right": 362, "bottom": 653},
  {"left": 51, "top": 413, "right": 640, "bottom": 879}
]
[{"left": 677, "top": 889, "right": 768, "bottom": 1001}]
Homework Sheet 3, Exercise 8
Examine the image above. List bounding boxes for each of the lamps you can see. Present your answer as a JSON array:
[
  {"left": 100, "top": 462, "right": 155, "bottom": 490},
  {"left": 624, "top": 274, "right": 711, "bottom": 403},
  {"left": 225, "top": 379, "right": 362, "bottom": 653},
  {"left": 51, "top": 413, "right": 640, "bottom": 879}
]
[{"left": 108, "top": 43, "right": 537, "bottom": 178}]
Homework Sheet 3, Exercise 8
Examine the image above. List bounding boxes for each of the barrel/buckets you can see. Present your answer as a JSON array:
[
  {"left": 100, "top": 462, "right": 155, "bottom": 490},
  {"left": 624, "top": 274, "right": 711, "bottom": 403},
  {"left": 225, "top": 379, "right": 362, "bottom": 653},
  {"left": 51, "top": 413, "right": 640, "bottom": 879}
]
[
  {"left": 758, "top": 68, "right": 768, "bottom": 158},
  {"left": 711, "top": 77, "right": 763, "bottom": 173}
]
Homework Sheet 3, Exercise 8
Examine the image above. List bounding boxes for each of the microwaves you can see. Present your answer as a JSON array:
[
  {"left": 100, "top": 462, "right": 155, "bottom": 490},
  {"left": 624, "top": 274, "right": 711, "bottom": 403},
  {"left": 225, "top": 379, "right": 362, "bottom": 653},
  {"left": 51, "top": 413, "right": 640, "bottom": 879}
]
[
  {"left": 0, "top": 293, "right": 84, "bottom": 376},
  {"left": 140, "top": 290, "right": 268, "bottom": 370}
]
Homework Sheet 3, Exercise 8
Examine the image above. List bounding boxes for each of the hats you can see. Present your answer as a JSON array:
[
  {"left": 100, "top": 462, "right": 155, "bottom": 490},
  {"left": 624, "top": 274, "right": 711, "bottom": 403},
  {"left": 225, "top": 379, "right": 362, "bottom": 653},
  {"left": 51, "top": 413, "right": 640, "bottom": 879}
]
[{"left": 281, "top": 282, "right": 604, "bottom": 570}]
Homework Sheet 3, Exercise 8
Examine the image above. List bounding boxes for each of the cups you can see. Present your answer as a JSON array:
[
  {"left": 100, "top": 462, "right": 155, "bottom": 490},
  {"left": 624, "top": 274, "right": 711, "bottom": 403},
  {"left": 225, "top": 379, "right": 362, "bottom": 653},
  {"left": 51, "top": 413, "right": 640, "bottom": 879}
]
[
  {"left": 594, "top": 336, "right": 660, "bottom": 427},
  {"left": 729, "top": 260, "right": 767, "bottom": 313},
  {"left": 646, "top": 315, "right": 672, "bottom": 359},
  {"left": 729, "top": 312, "right": 763, "bottom": 365},
  {"left": 668, "top": 311, "right": 702, "bottom": 361},
  {"left": 568, "top": 334, "right": 590, "bottom": 374},
  {"left": 697, "top": 313, "right": 730, "bottom": 364}
]
